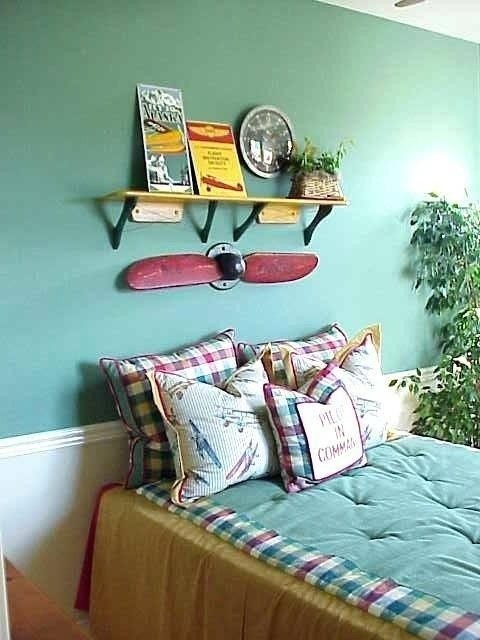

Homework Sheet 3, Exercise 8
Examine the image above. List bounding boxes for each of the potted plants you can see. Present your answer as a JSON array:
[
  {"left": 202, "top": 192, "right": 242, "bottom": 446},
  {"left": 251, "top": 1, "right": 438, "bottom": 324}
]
[{"left": 278, "top": 131, "right": 356, "bottom": 201}]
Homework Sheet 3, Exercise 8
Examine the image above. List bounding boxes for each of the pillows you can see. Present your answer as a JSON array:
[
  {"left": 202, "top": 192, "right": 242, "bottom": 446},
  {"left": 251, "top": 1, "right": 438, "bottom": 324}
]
[
  {"left": 100, "top": 328, "right": 237, "bottom": 491},
  {"left": 263, "top": 377, "right": 366, "bottom": 495},
  {"left": 236, "top": 321, "right": 355, "bottom": 389},
  {"left": 282, "top": 322, "right": 408, "bottom": 452},
  {"left": 149, "top": 349, "right": 282, "bottom": 505}
]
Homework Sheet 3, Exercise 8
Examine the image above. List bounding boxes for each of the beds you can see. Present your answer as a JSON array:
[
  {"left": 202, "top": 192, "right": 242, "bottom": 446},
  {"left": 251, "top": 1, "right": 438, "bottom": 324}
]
[{"left": 75, "top": 428, "right": 480, "bottom": 640}]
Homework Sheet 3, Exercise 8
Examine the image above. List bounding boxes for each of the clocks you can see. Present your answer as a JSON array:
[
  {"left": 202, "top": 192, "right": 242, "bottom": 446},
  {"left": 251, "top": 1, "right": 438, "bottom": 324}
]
[{"left": 238, "top": 104, "right": 297, "bottom": 178}]
